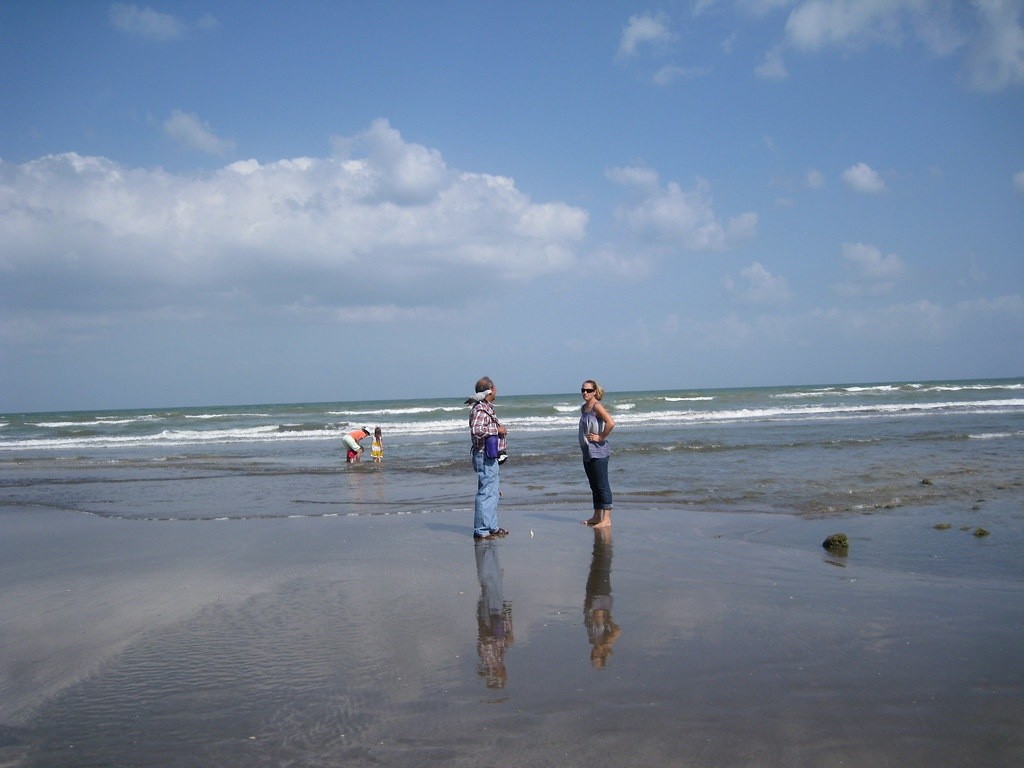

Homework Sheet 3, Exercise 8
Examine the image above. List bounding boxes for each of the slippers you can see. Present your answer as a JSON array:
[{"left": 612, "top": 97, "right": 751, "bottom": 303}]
[
  {"left": 496, "top": 528, "right": 508, "bottom": 535},
  {"left": 474, "top": 533, "right": 495, "bottom": 540}
]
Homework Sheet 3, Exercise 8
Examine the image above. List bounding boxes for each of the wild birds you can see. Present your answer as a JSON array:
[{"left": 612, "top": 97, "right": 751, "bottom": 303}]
[{"left": 465, "top": 390, "right": 493, "bottom": 405}]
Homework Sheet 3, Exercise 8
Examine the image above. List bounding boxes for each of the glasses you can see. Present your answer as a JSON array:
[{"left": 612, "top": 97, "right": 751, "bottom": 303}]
[{"left": 582, "top": 388, "right": 595, "bottom": 393}]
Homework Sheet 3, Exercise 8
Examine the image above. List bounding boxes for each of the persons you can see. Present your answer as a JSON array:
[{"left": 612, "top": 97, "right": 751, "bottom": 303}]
[
  {"left": 342, "top": 428, "right": 383, "bottom": 464},
  {"left": 469, "top": 377, "right": 509, "bottom": 542},
  {"left": 583, "top": 530, "right": 621, "bottom": 686},
  {"left": 475, "top": 543, "right": 515, "bottom": 689},
  {"left": 578, "top": 380, "right": 614, "bottom": 529}
]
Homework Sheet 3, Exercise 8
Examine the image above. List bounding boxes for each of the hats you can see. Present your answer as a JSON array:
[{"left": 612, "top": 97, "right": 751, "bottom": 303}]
[{"left": 363, "top": 427, "right": 371, "bottom": 436}]
